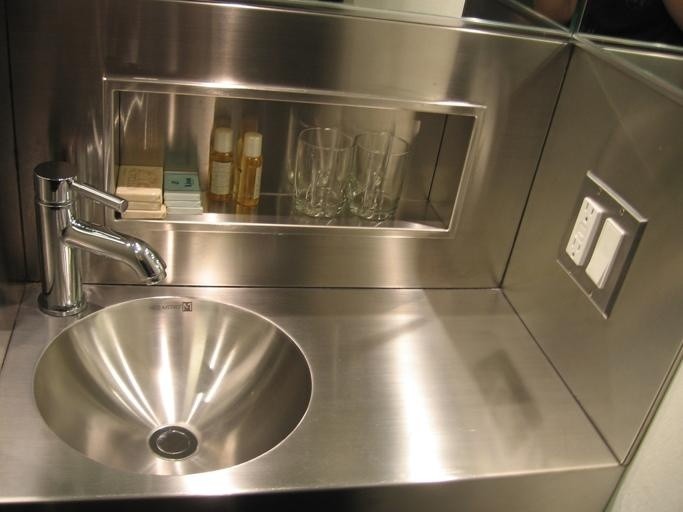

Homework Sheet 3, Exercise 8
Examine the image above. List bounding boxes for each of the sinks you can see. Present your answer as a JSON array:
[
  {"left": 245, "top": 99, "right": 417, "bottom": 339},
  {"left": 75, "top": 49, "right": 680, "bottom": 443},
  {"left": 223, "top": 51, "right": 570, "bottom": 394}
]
[{"left": 31, "top": 293, "right": 314, "bottom": 477}]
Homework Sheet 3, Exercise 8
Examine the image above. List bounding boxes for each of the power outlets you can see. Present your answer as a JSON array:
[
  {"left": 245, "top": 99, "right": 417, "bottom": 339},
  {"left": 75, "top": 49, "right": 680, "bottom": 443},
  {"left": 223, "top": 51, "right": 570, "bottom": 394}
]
[{"left": 555, "top": 171, "right": 648, "bottom": 319}]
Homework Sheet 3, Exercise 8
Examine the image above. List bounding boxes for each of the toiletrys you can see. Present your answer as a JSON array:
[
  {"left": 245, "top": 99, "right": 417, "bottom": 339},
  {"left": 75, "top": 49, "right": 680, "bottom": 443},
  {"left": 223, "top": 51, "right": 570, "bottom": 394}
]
[{"left": 205, "top": 127, "right": 234, "bottom": 202}]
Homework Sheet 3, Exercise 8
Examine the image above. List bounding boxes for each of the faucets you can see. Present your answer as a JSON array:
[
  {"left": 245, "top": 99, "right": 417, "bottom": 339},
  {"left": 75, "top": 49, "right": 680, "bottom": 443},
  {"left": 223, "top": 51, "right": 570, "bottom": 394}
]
[{"left": 32, "top": 159, "right": 165, "bottom": 317}]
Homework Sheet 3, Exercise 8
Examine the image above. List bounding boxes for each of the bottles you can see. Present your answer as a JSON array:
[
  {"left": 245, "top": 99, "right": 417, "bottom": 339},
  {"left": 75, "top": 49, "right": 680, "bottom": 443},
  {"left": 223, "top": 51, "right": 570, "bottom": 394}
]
[{"left": 209, "top": 127, "right": 236, "bottom": 202}]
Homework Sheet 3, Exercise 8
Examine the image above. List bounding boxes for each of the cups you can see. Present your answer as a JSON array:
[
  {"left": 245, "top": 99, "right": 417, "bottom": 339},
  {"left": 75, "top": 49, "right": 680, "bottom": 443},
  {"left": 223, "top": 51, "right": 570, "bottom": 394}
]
[
  {"left": 293, "top": 125, "right": 357, "bottom": 219},
  {"left": 347, "top": 131, "right": 413, "bottom": 222}
]
